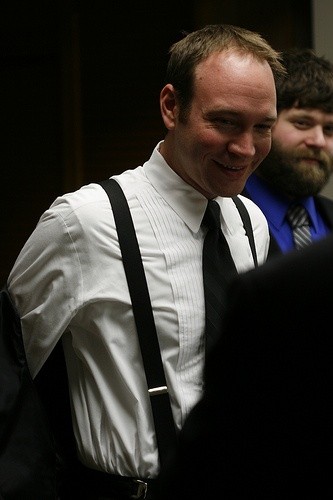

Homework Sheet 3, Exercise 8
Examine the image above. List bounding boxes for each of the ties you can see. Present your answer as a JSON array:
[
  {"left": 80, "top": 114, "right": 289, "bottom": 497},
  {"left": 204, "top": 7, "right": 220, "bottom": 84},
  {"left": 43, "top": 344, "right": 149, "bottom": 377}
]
[
  {"left": 200, "top": 200, "right": 239, "bottom": 395},
  {"left": 285, "top": 205, "right": 311, "bottom": 251}
]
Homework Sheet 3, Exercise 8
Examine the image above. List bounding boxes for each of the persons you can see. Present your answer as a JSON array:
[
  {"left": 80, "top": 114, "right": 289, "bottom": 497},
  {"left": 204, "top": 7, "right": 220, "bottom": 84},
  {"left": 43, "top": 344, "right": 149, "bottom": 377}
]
[
  {"left": 161, "top": 235, "right": 333, "bottom": 500},
  {"left": 243, "top": 46, "right": 333, "bottom": 256},
  {"left": 0, "top": 24, "right": 290, "bottom": 500}
]
